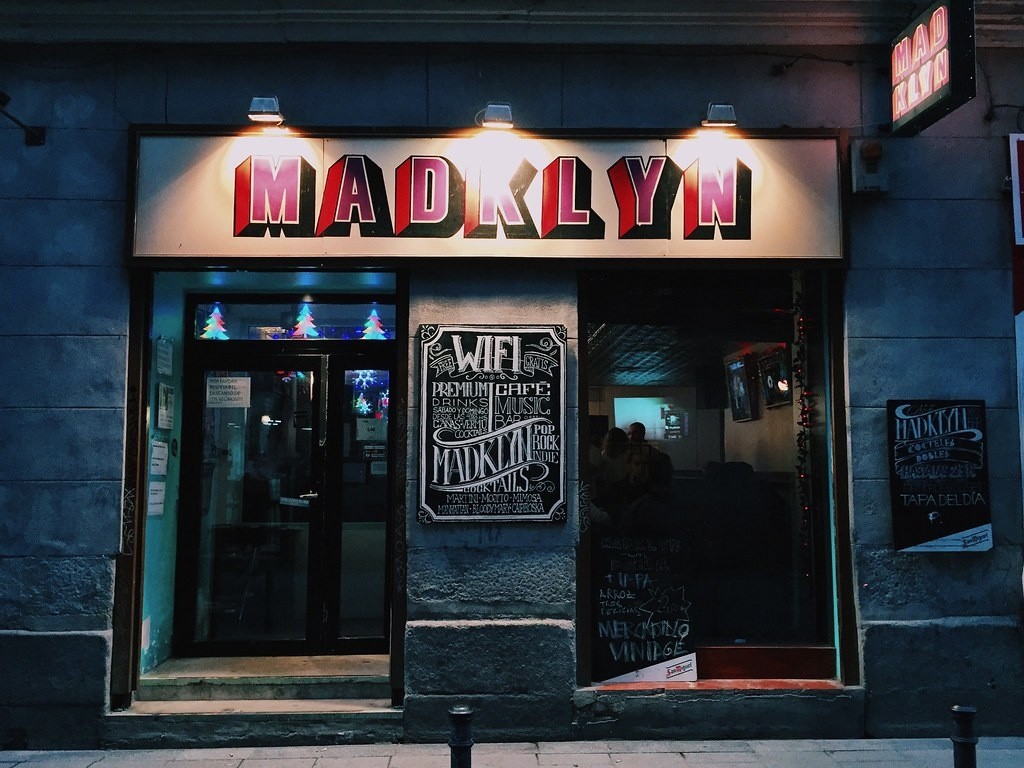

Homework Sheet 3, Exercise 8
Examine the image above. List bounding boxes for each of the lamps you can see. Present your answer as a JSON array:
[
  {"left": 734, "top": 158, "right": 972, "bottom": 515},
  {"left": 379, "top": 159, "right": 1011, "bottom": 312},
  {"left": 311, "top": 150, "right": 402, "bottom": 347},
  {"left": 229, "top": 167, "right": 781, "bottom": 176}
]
[
  {"left": 246, "top": 95, "right": 285, "bottom": 126},
  {"left": 474, "top": 101, "right": 514, "bottom": 129},
  {"left": 701, "top": 102, "right": 736, "bottom": 129}
]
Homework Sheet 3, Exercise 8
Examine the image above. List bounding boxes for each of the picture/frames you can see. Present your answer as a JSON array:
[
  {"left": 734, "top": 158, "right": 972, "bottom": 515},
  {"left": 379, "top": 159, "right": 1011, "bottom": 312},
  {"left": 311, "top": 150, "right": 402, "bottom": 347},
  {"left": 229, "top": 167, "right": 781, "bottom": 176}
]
[
  {"left": 723, "top": 355, "right": 753, "bottom": 424},
  {"left": 760, "top": 351, "right": 792, "bottom": 410}
]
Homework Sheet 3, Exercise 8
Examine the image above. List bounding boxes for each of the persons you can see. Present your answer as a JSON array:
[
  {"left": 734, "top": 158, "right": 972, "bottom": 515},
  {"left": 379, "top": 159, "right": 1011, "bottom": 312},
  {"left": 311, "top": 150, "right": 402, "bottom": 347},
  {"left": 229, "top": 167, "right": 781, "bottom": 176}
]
[{"left": 585, "top": 422, "right": 729, "bottom": 537}]
